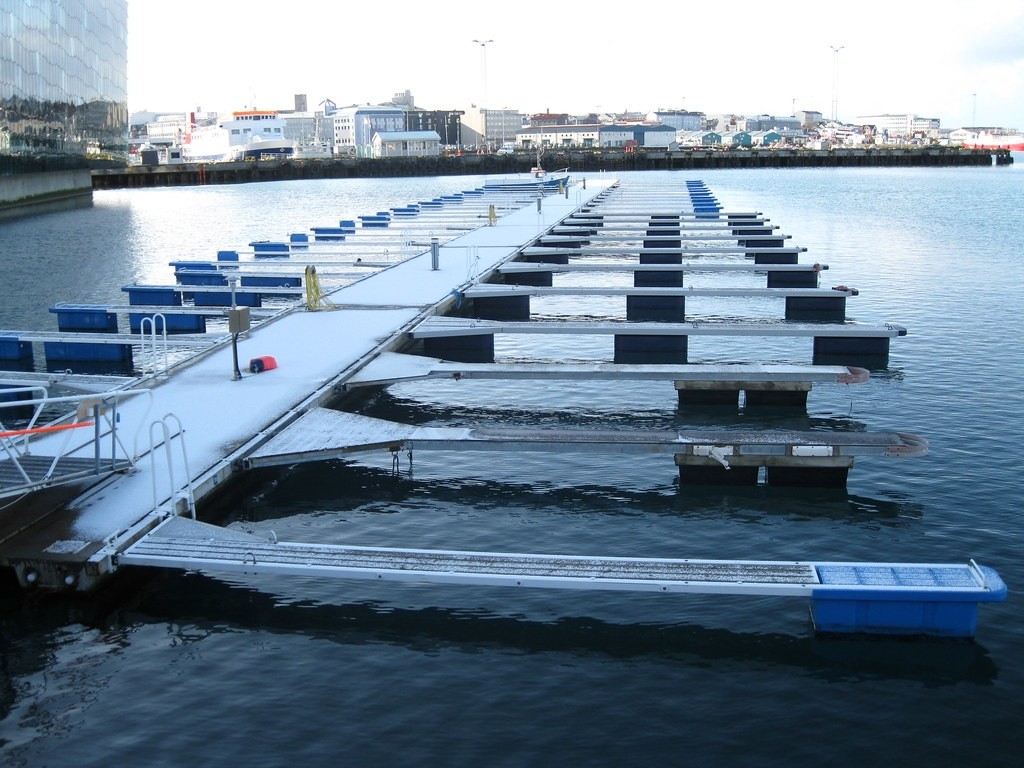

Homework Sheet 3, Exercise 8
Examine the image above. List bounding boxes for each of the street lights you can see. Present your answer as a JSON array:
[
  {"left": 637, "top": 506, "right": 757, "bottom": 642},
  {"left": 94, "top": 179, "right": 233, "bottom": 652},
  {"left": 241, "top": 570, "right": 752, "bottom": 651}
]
[
  {"left": 830, "top": 44, "right": 845, "bottom": 122},
  {"left": 471, "top": 38, "right": 494, "bottom": 103},
  {"left": 501, "top": 106, "right": 507, "bottom": 148}
]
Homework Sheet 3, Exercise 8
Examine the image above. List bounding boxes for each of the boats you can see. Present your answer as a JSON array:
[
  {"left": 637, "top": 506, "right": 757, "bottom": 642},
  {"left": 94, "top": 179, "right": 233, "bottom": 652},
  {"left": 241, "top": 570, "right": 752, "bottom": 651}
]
[
  {"left": 179, "top": 107, "right": 297, "bottom": 160},
  {"left": 529, "top": 166, "right": 547, "bottom": 175},
  {"left": 483, "top": 174, "right": 570, "bottom": 194}
]
